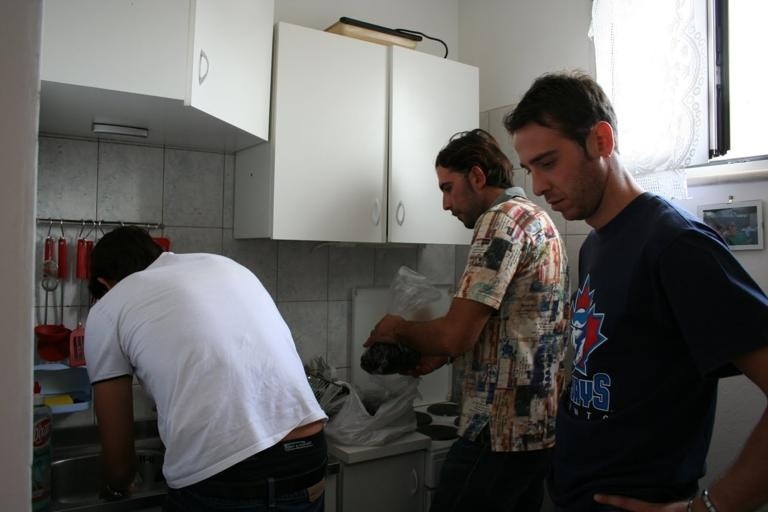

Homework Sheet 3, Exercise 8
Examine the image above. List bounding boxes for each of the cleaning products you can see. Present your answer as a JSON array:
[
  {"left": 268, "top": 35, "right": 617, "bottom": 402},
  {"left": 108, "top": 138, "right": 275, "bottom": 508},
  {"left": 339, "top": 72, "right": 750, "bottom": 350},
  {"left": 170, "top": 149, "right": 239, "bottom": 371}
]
[{"left": 32, "top": 382, "right": 53, "bottom": 512}]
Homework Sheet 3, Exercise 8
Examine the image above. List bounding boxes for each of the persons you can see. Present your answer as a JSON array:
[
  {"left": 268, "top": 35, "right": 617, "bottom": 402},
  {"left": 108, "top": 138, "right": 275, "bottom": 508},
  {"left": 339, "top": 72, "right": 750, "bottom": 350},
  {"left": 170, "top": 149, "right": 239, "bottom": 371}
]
[
  {"left": 80, "top": 225, "right": 331, "bottom": 512},
  {"left": 501, "top": 74, "right": 768, "bottom": 511},
  {"left": 360, "top": 128, "right": 571, "bottom": 512}
]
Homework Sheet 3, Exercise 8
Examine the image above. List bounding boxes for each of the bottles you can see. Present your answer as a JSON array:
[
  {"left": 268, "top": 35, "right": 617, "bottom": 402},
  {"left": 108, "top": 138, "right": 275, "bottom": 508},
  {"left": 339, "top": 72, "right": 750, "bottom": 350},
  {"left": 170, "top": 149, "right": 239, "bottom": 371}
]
[{"left": 28, "top": 380, "right": 55, "bottom": 512}]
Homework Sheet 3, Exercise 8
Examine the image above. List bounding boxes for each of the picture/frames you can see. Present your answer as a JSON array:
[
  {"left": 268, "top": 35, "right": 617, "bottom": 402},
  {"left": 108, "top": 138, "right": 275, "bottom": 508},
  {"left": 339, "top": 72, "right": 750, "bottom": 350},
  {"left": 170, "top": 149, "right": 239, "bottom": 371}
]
[{"left": 697, "top": 198, "right": 764, "bottom": 251}]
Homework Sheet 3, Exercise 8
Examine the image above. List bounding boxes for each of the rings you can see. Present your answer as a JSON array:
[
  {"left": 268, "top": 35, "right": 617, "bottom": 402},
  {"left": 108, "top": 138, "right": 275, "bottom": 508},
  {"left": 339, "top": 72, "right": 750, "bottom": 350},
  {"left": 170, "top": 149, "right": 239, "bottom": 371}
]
[{"left": 369, "top": 328, "right": 376, "bottom": 336}]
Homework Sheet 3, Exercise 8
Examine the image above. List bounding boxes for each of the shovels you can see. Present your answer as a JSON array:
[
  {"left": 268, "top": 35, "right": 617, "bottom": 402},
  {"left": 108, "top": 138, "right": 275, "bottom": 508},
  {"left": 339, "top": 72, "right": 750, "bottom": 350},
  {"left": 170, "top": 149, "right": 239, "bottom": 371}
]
[{"left": 70, "top": 238, "right": 85, "bottom": 366}]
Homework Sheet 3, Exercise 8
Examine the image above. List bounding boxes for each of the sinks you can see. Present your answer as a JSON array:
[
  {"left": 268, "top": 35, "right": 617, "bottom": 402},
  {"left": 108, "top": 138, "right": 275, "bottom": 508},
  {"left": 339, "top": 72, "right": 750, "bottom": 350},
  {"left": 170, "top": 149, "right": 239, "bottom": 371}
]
[{"left": 51, "top": 448, "right": 169, "bottom": 512}]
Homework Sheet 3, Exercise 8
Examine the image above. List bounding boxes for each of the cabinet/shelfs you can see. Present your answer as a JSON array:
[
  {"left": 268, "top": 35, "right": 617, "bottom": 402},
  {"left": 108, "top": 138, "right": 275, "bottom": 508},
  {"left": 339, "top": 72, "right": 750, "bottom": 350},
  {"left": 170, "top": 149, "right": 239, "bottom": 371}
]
[
  {"left": 340, "top": 449, "right": 448, "bottom": 512},
  {"left": 234, "top": 21, "right": 479, "bottom": 246}
]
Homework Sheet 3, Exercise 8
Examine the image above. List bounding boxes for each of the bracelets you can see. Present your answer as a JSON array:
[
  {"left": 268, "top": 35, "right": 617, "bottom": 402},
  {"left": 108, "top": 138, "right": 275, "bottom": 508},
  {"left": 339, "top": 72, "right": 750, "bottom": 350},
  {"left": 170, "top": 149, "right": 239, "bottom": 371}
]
[
  {"left": 700, "top": 485, "right": 718, "bottom": 512},
  {"left": 686, "top": 494, "right": 695, "bottom": 512}
]
